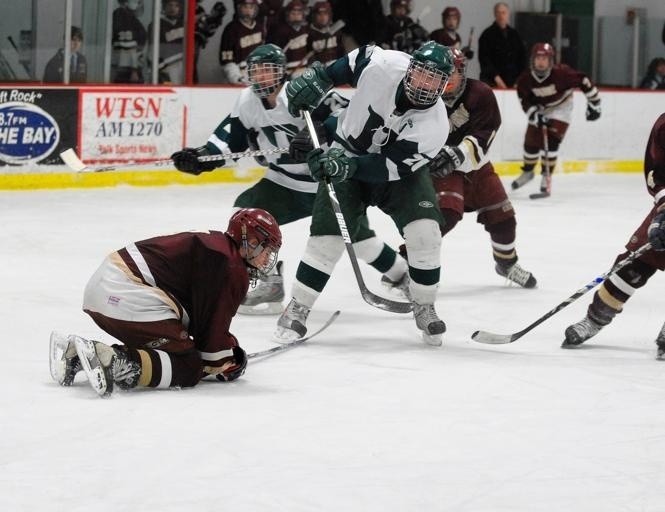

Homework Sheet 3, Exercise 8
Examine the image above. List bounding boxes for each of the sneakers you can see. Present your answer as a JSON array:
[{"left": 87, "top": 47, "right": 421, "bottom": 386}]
[
  {"left": 237, "top": 261, "right": 286, "bottom": 315},
  {"left": 656, "top": 324, "right": 665, "bottom": 360},
  {"left": 65, "top": 335, "right": 122, "bottom": 395},
  {"left": 494, "top": 262, "right": 537, "bottom": 289},
  {"left": 411, "top": 298, "right": 446, "bottom": 346},
  {"left": 381, "top": 272, "right": 413, "bottom": 302},
  {"left": 273, "top": 296, "right": 312, "bottom": 345},
  {"left": 561, "top": 316, "right": 603, "bottom": 350}
]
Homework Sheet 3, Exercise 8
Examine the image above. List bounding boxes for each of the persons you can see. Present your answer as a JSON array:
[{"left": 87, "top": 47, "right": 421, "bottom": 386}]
[
  {"left": 632, "top": 57, "right": 664, "bottom": 90},
  {"left": 562, "top": 111, "right": 664, "bottom": 350},
  {"left": 382, "top": 48, "right": 535, "bottom": 287},
  {"left": 478, "top": 2, "right": 525, "bottom": 89},
  {"left": 46, "top": 26, "right": 87, "bottom": 84},
  {"left": 278, "top": 40, "right": 456, "bottom": 336},
  {"left": 378, "top": 0, "right": 476, "bottom": 62},
  {"left": 110, "top": 1, "right": 263, "bottom": 87},
  {"left": 512, "top": 44, "right": 600, "bottom": 192},
  {"left": 63, "top": 206, "right": 282, "bottom": 399},
  {"left": 166, "top": 46, "right": 416, "bottom": 308},
  {"left": 274, "top": 1, "right": 360, "bottom": 66}
]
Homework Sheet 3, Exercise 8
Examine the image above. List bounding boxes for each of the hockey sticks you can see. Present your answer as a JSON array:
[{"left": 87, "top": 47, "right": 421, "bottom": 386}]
[
  {"left": 245, "top": 310, "right": 339, "bottom": 359},
  {"left": 471, "top": 241, "right": 656, "bottom": 345},
  {"left": 60, "top": 147, "right": 293, "bottom": 173},
  {"left": 529, "top": 115, "right": 551, "bottom": 199},
  {"left": 303, "top": 109, "right": 418, "bottom": 312}
]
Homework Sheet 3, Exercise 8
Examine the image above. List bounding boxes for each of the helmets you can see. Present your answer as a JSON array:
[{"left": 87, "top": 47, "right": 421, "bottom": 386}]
[
  {"left": 442, "top": 47, "right": 466, "bottom": 106},
  {"left": 247, "top": 43, "right": 286, "bottom": 98},
  {"left": 235, "top": 1, "right": 333, "bottom": 26},
  {"left": 403, "top": 42, "right": 454, "bottom": 107},
  {"left": 529, "top": 42, "right": 554, "bottom": 57},
  {"left": 391, "top": 0, "right": 411, "bottom": 17},
  {"left": 442, "top": 8, "right": 460, "bottom": 30}
]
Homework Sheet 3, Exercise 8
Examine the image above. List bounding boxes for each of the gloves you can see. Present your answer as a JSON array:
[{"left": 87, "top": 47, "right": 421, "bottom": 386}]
[
  {"left": 586, "top": 99, "right": 601, "bottom": 120},
  {"left": 171, "top": 147, "right": 216, "bottom": 175},
  {"left": 427, "top": 145, "right": 464, "bottom": 180},
  {"left": 528, "top": 109, "right": 551, "bottom": 130},
  {"left": 307, "top": 148, "right": 358, "bottom": 183},
  {"left": 283, "top": 61, "right": 333, "bottom": 118}
]
